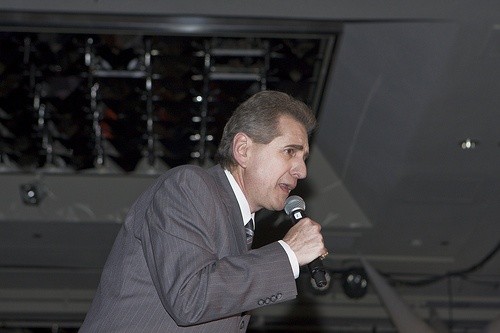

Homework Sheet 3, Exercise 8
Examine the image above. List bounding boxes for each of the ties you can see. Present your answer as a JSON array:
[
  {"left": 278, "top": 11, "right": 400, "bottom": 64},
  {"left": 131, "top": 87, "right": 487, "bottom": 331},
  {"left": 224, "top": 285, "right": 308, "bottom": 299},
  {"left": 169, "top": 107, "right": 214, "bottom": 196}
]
[{"left": 244, "top": 218, "right": 254, "bottom": 250}]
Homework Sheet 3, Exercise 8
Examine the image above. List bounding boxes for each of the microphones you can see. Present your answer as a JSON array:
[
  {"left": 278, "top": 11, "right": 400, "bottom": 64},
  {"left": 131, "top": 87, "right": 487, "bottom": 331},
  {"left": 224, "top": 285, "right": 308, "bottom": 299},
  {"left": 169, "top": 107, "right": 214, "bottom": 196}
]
[{"left": 283, "top": 194, "right": 327, "bottom": 287}]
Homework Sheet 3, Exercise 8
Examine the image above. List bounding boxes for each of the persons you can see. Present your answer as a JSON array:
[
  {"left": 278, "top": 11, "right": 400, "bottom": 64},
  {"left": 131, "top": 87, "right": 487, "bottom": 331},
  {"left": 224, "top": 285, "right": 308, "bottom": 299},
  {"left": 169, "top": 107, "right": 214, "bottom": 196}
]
[{"left": 77, "top": 90, "right": 329, "bottom": 333}]
